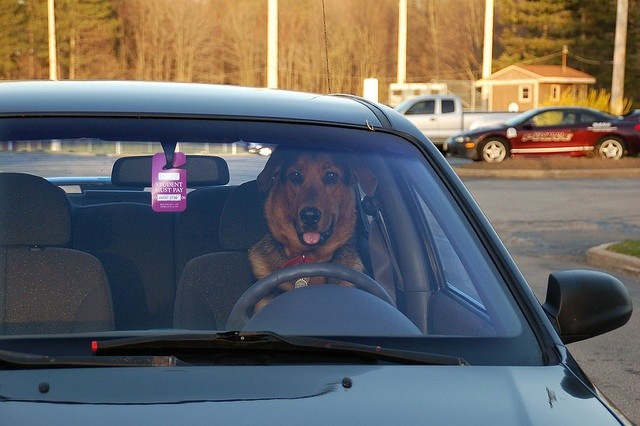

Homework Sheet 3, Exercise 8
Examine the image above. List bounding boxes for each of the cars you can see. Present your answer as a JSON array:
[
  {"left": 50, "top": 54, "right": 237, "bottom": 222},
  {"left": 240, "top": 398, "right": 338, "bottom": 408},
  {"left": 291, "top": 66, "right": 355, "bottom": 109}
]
[
  {"left": 1, "top": 80, "right": 636, "bottom": 426},
  {"left": 446, "top": 106, "right": 639, "bottom": 162}
]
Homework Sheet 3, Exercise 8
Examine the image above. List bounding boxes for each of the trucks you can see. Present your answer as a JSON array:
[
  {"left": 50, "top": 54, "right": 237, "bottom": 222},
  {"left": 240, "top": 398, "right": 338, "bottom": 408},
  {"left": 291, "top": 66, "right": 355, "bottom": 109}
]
[{"left": 396, "top": 96, "right": 525, "bottom": 150}]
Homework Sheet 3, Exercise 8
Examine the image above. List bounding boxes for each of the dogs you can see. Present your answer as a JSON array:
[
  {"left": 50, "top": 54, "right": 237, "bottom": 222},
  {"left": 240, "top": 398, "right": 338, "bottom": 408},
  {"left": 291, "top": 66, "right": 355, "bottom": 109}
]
[{"left": 251, "top": 140, "right": 387, "bottom": 318}]
